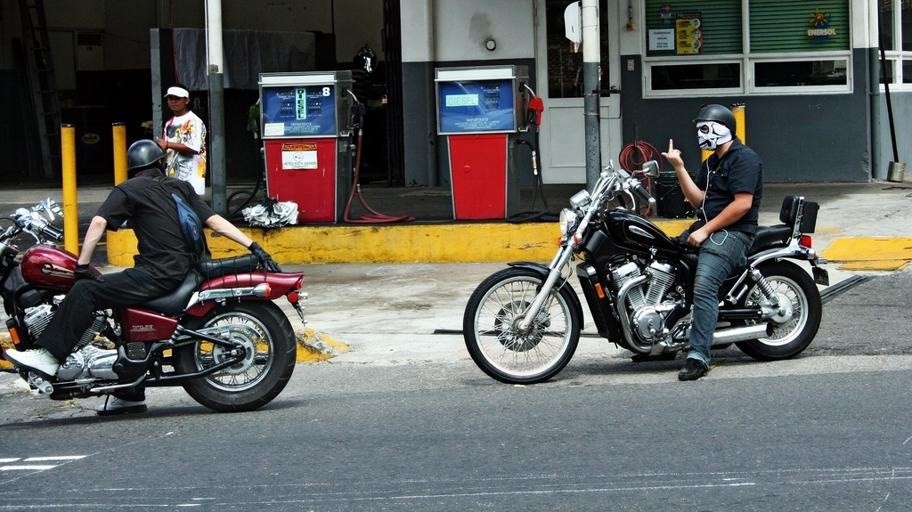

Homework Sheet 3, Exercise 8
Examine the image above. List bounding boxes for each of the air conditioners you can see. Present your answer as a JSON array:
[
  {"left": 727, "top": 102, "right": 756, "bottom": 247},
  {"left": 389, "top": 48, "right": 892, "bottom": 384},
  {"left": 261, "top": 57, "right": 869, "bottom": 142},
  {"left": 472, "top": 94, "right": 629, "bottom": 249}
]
[{"left": 74, "top": 29, "right": 106, "bottom": 72}]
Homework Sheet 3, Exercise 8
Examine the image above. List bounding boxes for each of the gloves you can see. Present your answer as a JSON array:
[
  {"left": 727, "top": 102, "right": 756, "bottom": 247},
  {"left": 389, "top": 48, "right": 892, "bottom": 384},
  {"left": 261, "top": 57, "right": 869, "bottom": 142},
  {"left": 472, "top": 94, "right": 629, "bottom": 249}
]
[
  {"left": 74, "top": 261, "right": 105, "bottom": 283},
  {"left": 248, "top": 241, "right": 282, "bottom": 273}
]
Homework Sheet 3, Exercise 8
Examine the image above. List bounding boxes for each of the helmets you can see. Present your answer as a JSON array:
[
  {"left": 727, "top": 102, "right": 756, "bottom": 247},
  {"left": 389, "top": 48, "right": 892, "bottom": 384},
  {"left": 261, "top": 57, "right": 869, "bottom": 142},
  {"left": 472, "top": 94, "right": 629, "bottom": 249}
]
[
  {"left": 127, "top": 138, "right": 165, "bottom": 171},
  {"left": 694, "top": 103, "right": 735, "bottom": 138}
]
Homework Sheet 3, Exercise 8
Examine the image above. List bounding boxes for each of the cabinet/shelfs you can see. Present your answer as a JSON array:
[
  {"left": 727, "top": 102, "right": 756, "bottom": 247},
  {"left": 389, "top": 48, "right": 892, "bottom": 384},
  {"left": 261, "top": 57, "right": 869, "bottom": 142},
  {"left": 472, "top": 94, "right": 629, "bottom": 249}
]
[
  {"left": 64, "top": 105, "right": 106, "bottom": 172},
  {"left": 76, "top": 68, "right": 151, "bottom": 173}
]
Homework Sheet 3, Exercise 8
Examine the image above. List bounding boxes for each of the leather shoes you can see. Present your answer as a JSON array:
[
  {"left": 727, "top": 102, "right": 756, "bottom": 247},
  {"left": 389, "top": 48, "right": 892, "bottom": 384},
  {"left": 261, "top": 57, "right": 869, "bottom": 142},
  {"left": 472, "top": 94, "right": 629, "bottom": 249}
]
[
  {"left": 2, "top": 347, "right": 61, "bottom": 382},
  {"left": 97, "top": 397, "right": 146, "bottom": 415},
  {"left": 631, "top": 351, "right": 676, "bottom": 361},
  {"left": 678, "top": 358, "right": 708, "bottom": 380}
]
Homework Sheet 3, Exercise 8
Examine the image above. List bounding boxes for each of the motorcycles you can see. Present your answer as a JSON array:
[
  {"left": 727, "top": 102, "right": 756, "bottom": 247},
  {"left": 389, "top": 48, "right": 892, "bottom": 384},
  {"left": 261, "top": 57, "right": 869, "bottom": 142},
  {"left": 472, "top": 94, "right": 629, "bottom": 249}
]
[
  {"left": 463, "top": 158, "right": 832, "bottom": 383},
  {"left": 0, "top": 197, "right": 309, "bottom": 415}
]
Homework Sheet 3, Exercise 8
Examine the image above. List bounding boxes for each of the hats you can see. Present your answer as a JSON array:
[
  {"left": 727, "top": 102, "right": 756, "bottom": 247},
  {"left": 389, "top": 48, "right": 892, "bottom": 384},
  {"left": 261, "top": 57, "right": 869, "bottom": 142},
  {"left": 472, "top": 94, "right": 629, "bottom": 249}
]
[{"left": 164, "top": 87, "right": 189, "bottom": 99}]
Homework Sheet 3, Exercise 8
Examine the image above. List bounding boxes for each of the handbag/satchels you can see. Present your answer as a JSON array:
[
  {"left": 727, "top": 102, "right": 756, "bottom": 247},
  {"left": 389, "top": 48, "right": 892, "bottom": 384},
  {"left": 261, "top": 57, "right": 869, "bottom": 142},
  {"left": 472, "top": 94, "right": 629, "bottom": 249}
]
[{"left": 153, "top": 180, "right": 213, "bottom": 262}]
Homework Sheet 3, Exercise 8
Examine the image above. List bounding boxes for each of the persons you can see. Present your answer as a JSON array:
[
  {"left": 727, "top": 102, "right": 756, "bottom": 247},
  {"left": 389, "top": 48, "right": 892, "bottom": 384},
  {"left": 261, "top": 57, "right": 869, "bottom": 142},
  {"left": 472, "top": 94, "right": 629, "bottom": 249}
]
[
  {"left": 631, "top": 103, "right": 762, "bottom": 382},
  {"left": 156, "top": 83, "right": 207, "bottom": 200},
  {"left": 5, "top": 140, "right": 282, "bottom": 418}
]
